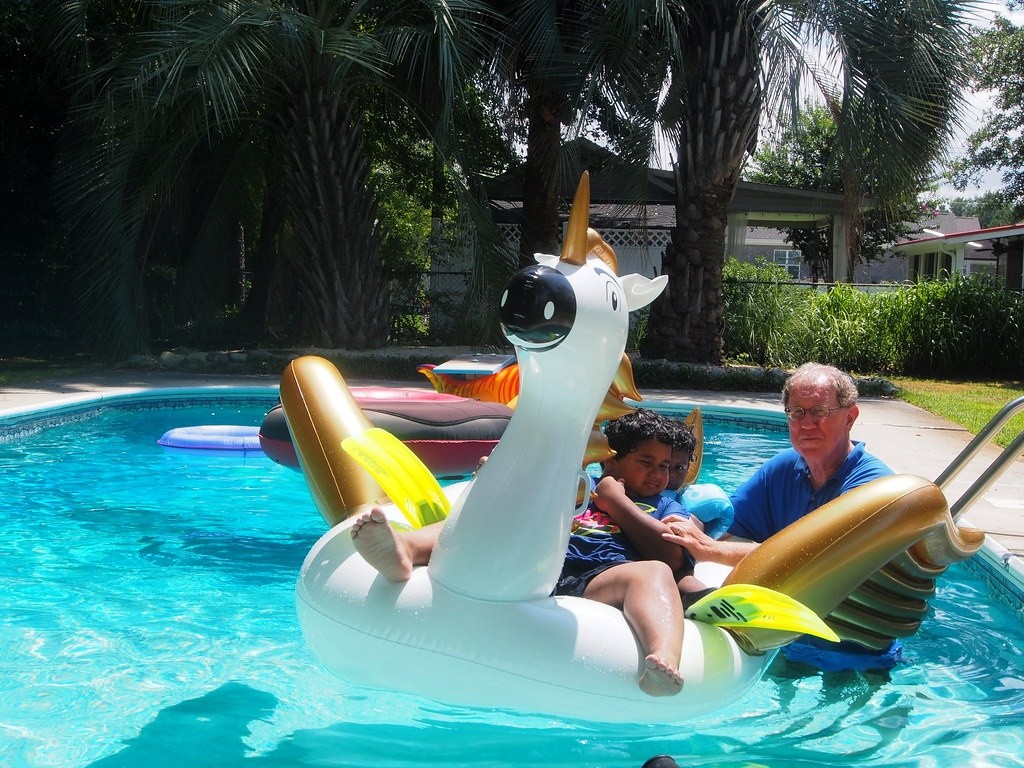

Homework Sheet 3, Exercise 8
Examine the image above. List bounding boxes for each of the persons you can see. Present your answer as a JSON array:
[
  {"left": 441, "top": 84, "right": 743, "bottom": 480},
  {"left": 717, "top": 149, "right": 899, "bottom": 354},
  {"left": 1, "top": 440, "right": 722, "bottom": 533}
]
[
  {"left": 349, "top": 407, "right": 705, "bottom": 697},
  {"left": 660, "top": 362, "right": 897, "bottom": 570}
]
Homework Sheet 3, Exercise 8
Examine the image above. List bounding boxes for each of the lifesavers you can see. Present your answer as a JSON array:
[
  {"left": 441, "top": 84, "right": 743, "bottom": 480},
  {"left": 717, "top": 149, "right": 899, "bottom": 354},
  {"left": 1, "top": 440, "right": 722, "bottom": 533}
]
[{"left": 155, "top": 423, "right": 272, "bottom": 460}]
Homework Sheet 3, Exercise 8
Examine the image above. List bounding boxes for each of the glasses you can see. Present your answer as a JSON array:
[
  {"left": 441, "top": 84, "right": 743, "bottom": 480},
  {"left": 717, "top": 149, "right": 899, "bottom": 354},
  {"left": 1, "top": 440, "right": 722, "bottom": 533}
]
[{"left": 783, "top": 403, "right": 848, "bottom": 421}]
